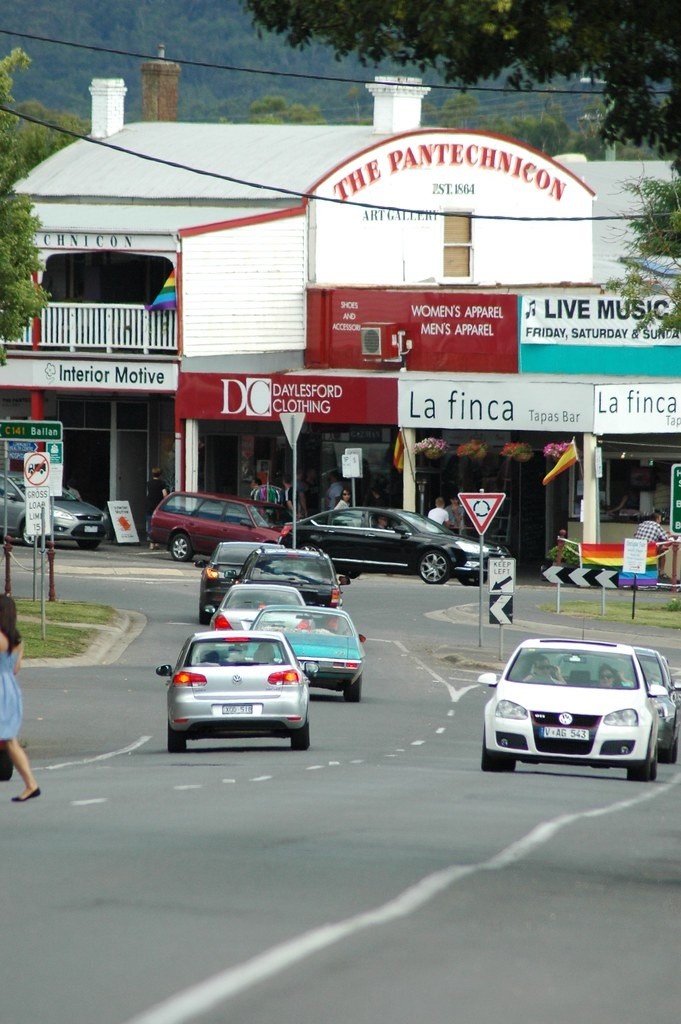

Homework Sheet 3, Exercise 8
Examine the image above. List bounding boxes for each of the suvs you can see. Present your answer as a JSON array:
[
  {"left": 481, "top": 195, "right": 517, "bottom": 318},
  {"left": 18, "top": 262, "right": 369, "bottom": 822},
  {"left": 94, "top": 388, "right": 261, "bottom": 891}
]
[{"left": 223, "top": 545, "right": 350, "bottom": 611}]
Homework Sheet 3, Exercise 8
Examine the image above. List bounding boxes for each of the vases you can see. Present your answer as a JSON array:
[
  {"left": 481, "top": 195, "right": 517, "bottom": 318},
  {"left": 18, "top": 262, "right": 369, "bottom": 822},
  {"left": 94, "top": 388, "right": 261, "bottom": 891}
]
[
  {"left": 426, "top": 449, "right": 441, "bottom": 459},
  {"left": 472, "top": 448, "right": 488, "bottom": 461},
  {"left": 515, "top": 452, "right": 532, "bottom": 462}
]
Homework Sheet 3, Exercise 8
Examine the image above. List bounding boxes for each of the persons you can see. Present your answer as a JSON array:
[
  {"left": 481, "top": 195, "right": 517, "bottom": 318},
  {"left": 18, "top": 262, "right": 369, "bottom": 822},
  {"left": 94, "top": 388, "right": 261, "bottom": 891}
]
[
  {"left": 524, "top": 656, "right": 566, "bottom": 684},
  {"left": 67, "top": 479, "right": 81, "bottom": 499},
  {"left": 376, "top": 516, "right": 388, "bottom": 528},
  {"left": 371, "top": 487, "right": 386, "bottom": 507},
  {"left": 326, "top": 470, "right": 351, "bottom": 524},
  {"left": 0, "top": 594, "right": 40, "bottom": 802},
  {"left": 282, "top": 469, "right": 318, "bottom": 519},
  {"left": 428, "top": 497, "right": 450, "bottom": 529},
  {"left": 445, "top": 496, "right": 463, "bottom": 535},
  {"left": 634, "top": 513, "right": 671, "bottom": 580},
  {"left": 600, "top": 664, "right": 634, "bottom": 687},
  {"left": 251, "top": 478, "right": 262, "bottom": 489},
  {"left": 147, "top": 468, "right": 168, "bottom": 549}
]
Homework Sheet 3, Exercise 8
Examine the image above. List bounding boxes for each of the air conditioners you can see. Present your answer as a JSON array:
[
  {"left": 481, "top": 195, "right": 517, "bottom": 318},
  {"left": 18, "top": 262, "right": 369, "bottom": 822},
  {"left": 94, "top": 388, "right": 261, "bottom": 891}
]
[{"left": 361, "top": 322, "right": 404, "bottom": 363}]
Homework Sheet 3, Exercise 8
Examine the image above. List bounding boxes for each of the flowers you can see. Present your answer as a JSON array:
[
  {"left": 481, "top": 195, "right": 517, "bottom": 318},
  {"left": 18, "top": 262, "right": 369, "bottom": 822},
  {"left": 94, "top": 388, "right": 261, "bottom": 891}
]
[
  {"left": 456, "top": 437, "right": 488, "bottom": 456},
  {"left": 500, "top": 440, "right": 533, "bottom": 458},
  {"left": 414, "top": 436, "right": 448, "bottom": 455},
  {"left": 543, "top": 441, "right": 570, "bottom": 458}
]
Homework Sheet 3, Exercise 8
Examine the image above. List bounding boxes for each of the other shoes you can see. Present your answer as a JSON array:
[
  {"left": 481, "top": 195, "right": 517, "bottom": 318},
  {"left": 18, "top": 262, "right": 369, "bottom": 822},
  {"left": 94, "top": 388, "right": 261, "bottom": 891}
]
[
  {"left": 150, "top": 543, "right": 158, "bottom": 551},
  {"left": 11, "top": 787, "right": 42, "bottom": 802}
]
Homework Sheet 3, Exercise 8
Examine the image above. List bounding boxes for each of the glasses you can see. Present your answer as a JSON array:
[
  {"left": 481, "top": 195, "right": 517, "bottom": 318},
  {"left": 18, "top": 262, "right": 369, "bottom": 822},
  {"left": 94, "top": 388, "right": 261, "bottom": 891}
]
[{"left": 343, "top": 492, "right": 350, "bottom": 495}]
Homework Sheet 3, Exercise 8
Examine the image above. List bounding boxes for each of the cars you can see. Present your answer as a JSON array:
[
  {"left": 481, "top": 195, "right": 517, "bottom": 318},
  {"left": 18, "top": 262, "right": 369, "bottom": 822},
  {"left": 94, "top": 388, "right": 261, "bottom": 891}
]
[
  {"left": 150, "top": 491, "right": 294, "bottom": 563},
  {"left": 277, "top": 507, "right": 511, "bottom": 587},
  {"left": 478, "top": 637, "right": 668, "bottom": 782},
  {"left": 156, "top": 629, "right": 320, "bottom": 753},
  {"left": 552, "top": 644, "right": 681, "bottom": 765},
  {"left": 247, "top": 604, "right": 366, "bottom": 702},
  {"left": 204, "top": 583, "right": 317, "bottom": 632},
  {"left": 0, "top": 469, "right": 109, "bottom": 550},
  {"left": 192, "top": 541, "right": 287, "bottom": 627}
]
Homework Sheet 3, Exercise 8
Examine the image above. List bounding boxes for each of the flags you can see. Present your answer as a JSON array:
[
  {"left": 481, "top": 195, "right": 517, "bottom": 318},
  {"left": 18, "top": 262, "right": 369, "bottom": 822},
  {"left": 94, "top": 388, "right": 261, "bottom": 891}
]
[
  {"left": 394, "top": 431, "right": 405, "bottom": 472},
  {"left": 146, "top": 270, "right": 176, "bottom": 311}
]
